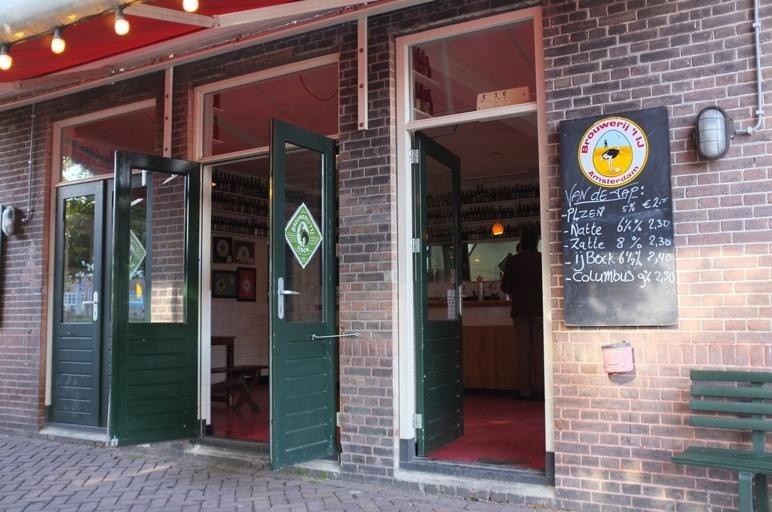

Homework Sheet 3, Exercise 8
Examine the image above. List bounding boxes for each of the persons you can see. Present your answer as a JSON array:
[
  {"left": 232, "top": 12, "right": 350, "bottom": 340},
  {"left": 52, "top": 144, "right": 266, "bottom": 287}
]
[
  {"left": 501, "top": 230, "right": 544, "bottom": 400},
  {"left": 75, "top": 273, "right": 93, "bottom": 316}
]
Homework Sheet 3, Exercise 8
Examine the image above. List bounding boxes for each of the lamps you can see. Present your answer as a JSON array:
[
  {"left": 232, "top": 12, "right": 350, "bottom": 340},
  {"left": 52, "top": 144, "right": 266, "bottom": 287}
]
[
  {"left": 0, "top": 8, "right": 130, "bottom": 73},
  {"left": 489, "top": 171, "right": 504, "bottom": 237},
  {"left": 689, "top": 106, "right": 736, "bottom": 161}
]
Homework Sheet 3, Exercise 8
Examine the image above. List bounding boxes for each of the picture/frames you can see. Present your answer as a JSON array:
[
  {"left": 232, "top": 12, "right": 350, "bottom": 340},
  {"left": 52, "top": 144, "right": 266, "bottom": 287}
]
[{"left": 211, "top": 235, "right": 259, "bottom": 302}]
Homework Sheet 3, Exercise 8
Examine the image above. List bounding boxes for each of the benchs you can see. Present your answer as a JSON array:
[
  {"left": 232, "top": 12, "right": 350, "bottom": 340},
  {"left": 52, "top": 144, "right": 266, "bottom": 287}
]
[{"left": 211, "top": 364, "right": 269, "bottom": 411}]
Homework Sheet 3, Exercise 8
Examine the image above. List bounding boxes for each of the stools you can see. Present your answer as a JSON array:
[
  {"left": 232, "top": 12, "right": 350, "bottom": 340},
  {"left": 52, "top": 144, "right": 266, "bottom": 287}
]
[{"left": 672, "top": 365, "right": 772, "bottom": 508}]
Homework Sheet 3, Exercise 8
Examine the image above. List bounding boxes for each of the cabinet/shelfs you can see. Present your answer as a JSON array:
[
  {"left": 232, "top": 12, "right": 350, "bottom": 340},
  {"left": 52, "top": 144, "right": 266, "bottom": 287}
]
[
  {"left": 411, "top": 67, "right": 440, "bottom": 118},
  {"left": 213, "top": 182, "right": 321, "bottom": 225},
  {"left": 424, "top": 196, "right": 541, "bottom": 245}
]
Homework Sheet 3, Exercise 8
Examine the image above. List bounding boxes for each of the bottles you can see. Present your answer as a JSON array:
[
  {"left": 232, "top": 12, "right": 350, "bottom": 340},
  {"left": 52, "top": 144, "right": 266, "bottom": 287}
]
[
  {"left": 425, "top": 182, "right": 539, "bottom": 242},
  {"left": 212, "top": 168, "right": 270, "bottom": 237}
]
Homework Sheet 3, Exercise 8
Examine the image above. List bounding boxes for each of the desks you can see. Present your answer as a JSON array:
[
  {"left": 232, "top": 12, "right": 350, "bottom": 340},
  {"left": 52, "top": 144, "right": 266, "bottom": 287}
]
[{"left": 211, "top": 333, "right": 237, "bottom": 407}]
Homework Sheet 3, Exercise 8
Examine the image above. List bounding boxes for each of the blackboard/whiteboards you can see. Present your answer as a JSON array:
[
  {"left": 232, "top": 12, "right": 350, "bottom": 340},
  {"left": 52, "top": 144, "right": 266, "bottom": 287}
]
[{"left": 558, "top": 106, "right": 678, "bottom": 327}]
